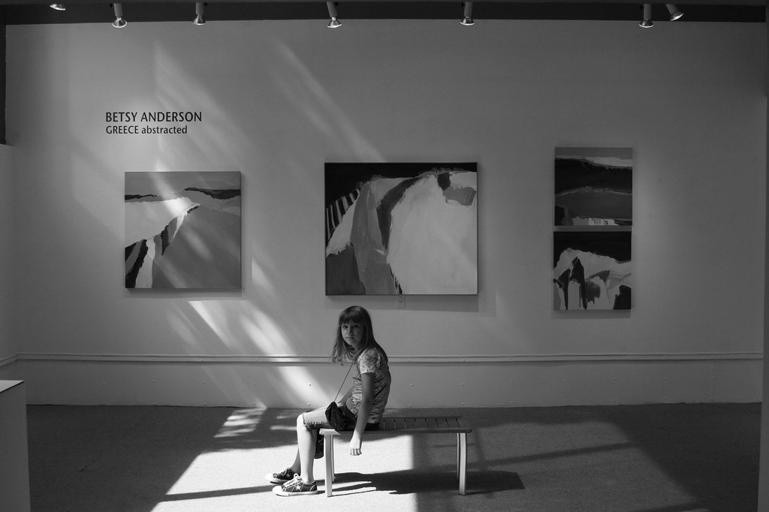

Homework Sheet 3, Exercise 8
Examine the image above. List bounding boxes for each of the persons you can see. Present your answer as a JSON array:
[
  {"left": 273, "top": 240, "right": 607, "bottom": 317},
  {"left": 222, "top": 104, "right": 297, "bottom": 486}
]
[{"left": 267, "top": 304, "right": 393, "bottom": 498}]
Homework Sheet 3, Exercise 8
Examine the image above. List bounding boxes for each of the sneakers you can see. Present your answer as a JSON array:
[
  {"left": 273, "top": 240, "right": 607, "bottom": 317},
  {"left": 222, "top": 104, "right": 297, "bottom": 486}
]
[
  {"left": 272, "top": 476, "right": 318, "bottom": 497},
  {"left": 267, "top": 468, "right": 300, "bottom": 483}
]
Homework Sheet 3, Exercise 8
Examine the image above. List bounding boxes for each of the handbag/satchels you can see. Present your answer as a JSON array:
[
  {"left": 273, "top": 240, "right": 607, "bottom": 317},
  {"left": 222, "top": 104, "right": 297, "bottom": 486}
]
[{"left": 325, "top": 401, "right": 349, "bottom": 431}]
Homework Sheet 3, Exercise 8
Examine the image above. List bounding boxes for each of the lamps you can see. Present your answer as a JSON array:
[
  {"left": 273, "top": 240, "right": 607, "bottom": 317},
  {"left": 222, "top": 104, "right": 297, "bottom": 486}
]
[
  {"left": 456, "top": 3, "right": 477, "bottom": 27},
  {"left": 639, "top": 3, "right": 658, "bottom": 29},
  {"left": 324, "top": 1, "right": 343, "bottom": 30},
  {"left": 111, "top": 2, "right": 130, "bottom": 29},
  {"left": 667, "top": 2, "right": 685, "bottom": 22},
  {"left": 48, "top": 1, "right": 69, "bottom": 12},
  {"left": 192, "top": 1, "right": 207, "bottom": 27}
]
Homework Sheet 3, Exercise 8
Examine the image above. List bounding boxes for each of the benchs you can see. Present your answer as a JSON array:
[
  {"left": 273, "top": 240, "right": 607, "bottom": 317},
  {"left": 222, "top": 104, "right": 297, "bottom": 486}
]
[{"left": 317, "top": 415, "right": 473, "bottom": 496}]
